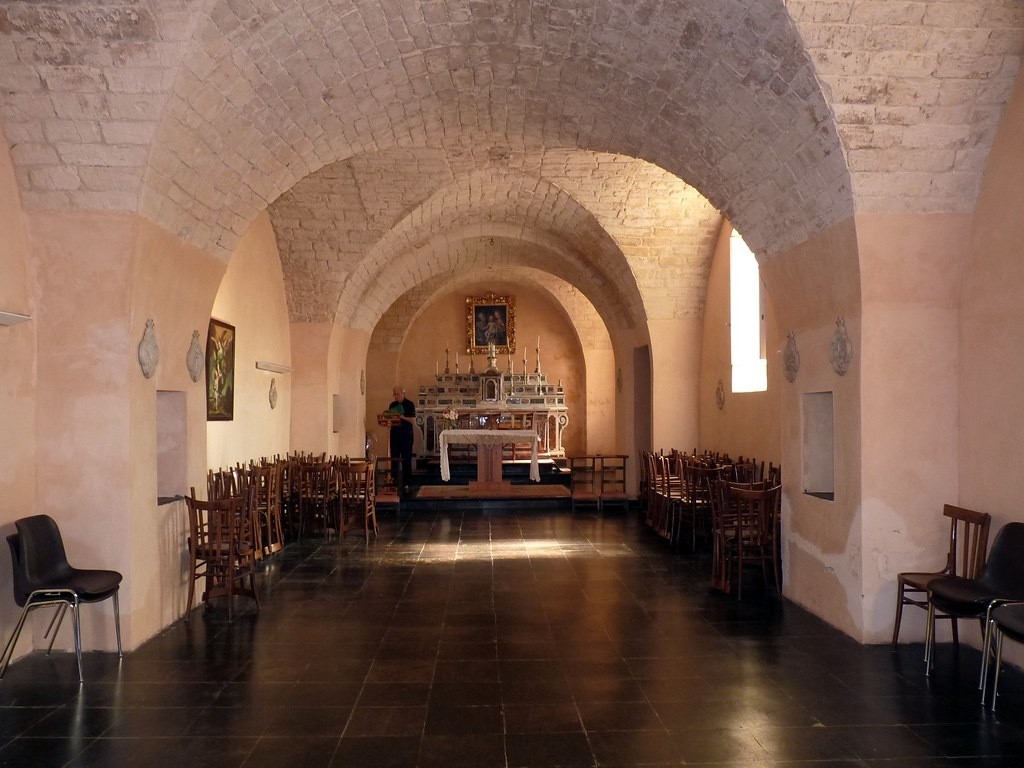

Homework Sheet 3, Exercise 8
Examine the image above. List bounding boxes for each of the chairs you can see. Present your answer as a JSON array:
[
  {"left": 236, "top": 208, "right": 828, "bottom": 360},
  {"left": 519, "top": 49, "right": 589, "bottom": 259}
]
[
  {"left": 567, "top": 454, "right": 599, "bottom": 513},
  {"left": 598, "top": 454, "right": 630, "bottom": 514},
  {"left": 0, "top": 514, "right": 123, "bottom": 681},
  {"left": 638, "top": 444, "right": 792, "bottom": 600},
  {"left": 184, "top": 448, "right": 411, "bottom": 623},
  {"left": 886, "top": 503, "right": 1024, "bottom": 712}
]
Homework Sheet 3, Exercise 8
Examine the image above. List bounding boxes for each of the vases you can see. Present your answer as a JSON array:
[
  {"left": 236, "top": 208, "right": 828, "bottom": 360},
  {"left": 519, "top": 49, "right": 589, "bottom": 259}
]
[{"left": 448, "top": 418, "right": 456, "bottom": 429}]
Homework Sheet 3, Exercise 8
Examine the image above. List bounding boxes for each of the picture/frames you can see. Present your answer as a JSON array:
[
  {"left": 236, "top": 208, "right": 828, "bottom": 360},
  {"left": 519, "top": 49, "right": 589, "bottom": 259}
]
[
  {"left": 206, "top": 318, "right": 235, "bottom": 422},
  {"left": 464, "top": 292, "right": 517, "bottom": 355}
]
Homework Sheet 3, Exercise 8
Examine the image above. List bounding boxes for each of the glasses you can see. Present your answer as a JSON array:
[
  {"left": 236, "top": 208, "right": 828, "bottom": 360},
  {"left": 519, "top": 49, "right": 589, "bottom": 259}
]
[{"left": 393, "top": 391, "right": 401, "bottom": 396}]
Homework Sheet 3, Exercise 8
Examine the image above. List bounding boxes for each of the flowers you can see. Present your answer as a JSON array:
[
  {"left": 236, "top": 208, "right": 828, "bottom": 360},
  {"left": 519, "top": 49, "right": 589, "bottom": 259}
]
[{"left": 442, "top": 406, "right": 458, "bottom": 421}]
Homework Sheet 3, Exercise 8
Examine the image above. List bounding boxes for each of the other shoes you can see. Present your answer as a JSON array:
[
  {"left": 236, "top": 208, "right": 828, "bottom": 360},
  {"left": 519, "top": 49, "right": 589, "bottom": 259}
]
[{"left": 403, "top": 485, "right": 408, "bottom": 488}]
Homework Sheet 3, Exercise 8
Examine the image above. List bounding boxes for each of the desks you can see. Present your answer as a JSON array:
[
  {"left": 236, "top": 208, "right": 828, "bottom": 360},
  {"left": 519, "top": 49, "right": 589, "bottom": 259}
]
[{"left": 438, "top": 428, "right": 541, "bottom": 492}]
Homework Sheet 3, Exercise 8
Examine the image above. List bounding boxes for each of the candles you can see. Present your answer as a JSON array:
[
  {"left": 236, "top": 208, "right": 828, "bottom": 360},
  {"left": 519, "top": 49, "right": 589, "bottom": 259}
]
[{"left": 435, "top": 336, "right": 544, "bottom": 378}]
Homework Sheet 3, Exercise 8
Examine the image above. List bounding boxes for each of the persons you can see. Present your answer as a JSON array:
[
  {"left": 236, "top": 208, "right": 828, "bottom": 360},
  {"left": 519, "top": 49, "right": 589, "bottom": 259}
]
[{"left": 388, "top": 386, "right": 416, "bottom": 487}]
